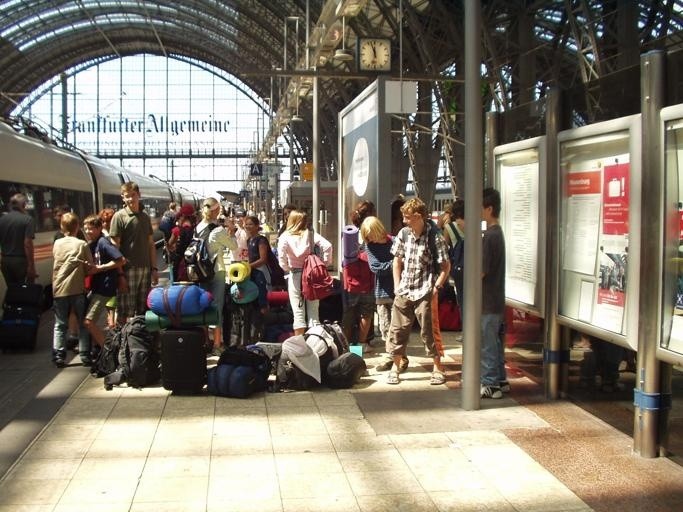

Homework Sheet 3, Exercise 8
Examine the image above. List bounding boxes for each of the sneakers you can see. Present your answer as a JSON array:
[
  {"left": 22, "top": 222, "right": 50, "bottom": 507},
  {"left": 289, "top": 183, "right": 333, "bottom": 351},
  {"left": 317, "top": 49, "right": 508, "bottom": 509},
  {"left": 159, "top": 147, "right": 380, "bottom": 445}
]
[
  {"left": 479, "top": 385, "right": 502, "bottom": 399},
  {"left": 499, "top": 380, "right": 510, "bottom": 393},
  {"left": 375, "top": 358, "right": 392, "bottom": 371},
  {"left": 203, "top": 341, "right": 213, "bottom": 353},
  {"left": 50, "top": 339, "right": 100, "bottom": 368},
  {"left": 356, "top": 342, "right": 375, "bottom": 352},
  {"left": 211, "top": 344, "right": 227, "bottom": 356},
  {"left": 397, "top": 357, "right": 408, "bottom": 374}
]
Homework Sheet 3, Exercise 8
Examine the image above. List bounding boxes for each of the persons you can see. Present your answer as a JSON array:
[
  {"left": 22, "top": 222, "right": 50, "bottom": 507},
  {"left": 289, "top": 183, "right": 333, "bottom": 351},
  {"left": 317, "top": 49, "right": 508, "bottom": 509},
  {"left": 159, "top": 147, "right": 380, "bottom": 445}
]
[
  {"left": 53, "top": 183, "right": 463, "bottom": 387},
  {"left": 481, "top": 187, "right": 511, "bottom": 400},
  {"left": 0, "top": 192, "right": 39, "bottom": 289},
  {"left": 587, "top": 335, "right": 638, "bottom": 394},
  {"left": 40, "top": 209, "right": 53, "bottom": 231}
]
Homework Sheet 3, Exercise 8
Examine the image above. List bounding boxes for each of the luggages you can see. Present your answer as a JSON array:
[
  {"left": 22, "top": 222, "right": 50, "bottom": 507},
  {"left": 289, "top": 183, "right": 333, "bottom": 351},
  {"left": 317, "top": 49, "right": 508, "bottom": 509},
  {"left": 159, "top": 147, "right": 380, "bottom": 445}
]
[{"left": 159, "top": 326, "right": 207, "bottom": 392}]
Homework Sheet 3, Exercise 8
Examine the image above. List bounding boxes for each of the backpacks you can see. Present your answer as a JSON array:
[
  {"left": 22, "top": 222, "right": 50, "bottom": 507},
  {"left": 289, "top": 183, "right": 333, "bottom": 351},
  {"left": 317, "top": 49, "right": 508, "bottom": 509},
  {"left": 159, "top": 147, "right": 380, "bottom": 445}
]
[
  {"left": 449, "top": 222, "right": 463, "bottom": 282},
  {"left": 247, "top": 237, "right": 285, "bottom": 287},
  {"left": 343, "top": 241, "right": 375, "bottom": 294},
  {"left": 183, "top": 223, "right": 218, "bottom": 283},
  {"left": 176, "top": 224, "right": 197, "bottom": 255},
  {"left": 298, "top": 229, "right": 334, "bottom": 308},
  {"left": 103, "top": 316, "right": 159, "bottom": 391}
]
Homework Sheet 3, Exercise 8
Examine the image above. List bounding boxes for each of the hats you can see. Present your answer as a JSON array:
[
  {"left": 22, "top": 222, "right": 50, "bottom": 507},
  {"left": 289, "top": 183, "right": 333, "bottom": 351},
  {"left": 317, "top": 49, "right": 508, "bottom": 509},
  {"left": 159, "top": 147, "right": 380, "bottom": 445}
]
[
  {"left": 236, "top": 209, "right": 246, "bottom": 216},
  {"left": 181, "top": 204, "right": 195, "bottom": 217}
]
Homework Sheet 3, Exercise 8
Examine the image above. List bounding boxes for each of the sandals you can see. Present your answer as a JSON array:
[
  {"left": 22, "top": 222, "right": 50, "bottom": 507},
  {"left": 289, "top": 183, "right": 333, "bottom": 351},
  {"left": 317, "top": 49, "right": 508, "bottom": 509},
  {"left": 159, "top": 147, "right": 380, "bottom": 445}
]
[
  {"left": 386, "top": 371, "right": 399, "bottom": 384},
  {"left": 430, "top": 369, "right": 445, "bottom": 385}
]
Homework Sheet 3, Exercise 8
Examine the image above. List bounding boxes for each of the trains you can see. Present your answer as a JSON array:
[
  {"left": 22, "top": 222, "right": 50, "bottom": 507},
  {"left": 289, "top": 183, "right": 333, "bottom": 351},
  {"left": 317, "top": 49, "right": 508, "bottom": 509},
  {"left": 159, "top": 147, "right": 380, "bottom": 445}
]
[
  {"left": 401, "top": 175, "right": 455, "bottom": 226},
  {"left": 0, "top": 115, "right": 215, "bottom": 343}
]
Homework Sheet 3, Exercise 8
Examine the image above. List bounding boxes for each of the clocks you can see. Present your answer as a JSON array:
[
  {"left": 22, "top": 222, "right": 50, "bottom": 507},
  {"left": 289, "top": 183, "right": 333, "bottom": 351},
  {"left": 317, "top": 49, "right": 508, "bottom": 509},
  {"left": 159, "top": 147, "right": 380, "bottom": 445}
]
[{"left": 355, "top": 36, "right": 393, "bottom": 75}]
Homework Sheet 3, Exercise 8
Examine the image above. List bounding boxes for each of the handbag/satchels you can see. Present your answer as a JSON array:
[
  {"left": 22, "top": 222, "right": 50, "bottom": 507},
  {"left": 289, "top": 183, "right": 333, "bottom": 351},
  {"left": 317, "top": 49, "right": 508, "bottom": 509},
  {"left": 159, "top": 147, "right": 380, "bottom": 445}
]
[
  {"left": 305, "top": 319, "right": 350, "bottom": 363},
  {"left": 3, "top": 307, "right": 38, "bottom": 320},
  {"left": 5, "top": 280, "right": 44, "bottom": 307},
  {"left": 0, "top": 320, "right": 37, "bottom": 351}
]
